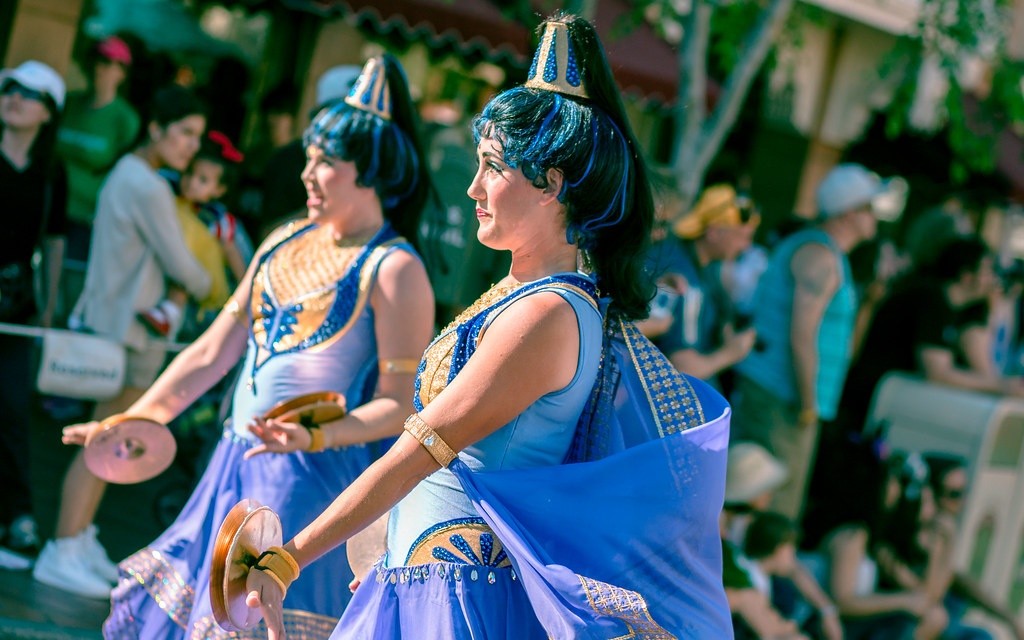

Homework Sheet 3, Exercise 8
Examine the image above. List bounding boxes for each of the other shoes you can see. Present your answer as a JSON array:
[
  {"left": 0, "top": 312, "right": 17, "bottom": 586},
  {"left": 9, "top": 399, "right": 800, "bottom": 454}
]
[{"left": 135, "top": 306, "right": 169, "bottom": 338}]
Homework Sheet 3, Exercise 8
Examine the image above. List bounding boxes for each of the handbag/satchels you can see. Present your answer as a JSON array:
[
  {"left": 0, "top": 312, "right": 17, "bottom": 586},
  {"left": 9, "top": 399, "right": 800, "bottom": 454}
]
[{"left": 36, "top": 330, "right": 127, "bottom": 400}]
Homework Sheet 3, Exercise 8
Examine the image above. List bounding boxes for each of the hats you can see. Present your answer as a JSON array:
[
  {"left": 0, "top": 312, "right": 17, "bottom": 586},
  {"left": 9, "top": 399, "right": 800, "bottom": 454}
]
[
  {"left": 672, "top": 183, "right": 762, "bottom": 238},
  {"left": 85, "top": 37, "right": 130, "bottom": 67},
  {"left": 818, "top": 164, "right": 894, "bottom": 214},
  {"left": 0, "top": 58, "right": 66, "bottom": 114},
  {"left": 722, "top": 440, "right": 792, "bottom": 504}
]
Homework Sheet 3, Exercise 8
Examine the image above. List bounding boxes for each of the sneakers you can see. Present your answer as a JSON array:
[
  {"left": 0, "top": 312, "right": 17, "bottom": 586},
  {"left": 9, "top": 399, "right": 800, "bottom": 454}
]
[
  {"left": 74, "top": 523, "right": 119, "bottom": 585},
  {"left": 32, "top": 537, "right": 114, "bottom": 598}
]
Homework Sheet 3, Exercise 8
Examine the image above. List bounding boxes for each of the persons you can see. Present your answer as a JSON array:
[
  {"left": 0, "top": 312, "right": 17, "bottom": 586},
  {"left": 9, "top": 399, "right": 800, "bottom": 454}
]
[
  {"left": 421, "top": 58, "right": 513, "bottom": 315},
  {"left": 627, "top": 166, "right": 1024, "bottom": 640},
  {"left": 62, "top": 54, "right": 449, "bottom": 640},
  {"left": 0, "top": 36, "right": 364, "bottom": 599},
  {"left": 247, "top": 15, "right": 732, "bottom": 640}
]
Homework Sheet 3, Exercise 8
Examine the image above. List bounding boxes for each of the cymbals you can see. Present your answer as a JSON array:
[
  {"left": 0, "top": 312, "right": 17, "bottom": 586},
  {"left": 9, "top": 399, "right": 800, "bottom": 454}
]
[
  {"left": 263, "top": 391, "right": 346, "bottom": 431},
  {"left": 207, "top": 497, "right": 284, "bottom": 633},
  {"left": 83, "top": 414, "right": 178, "bottom": 486}
]
